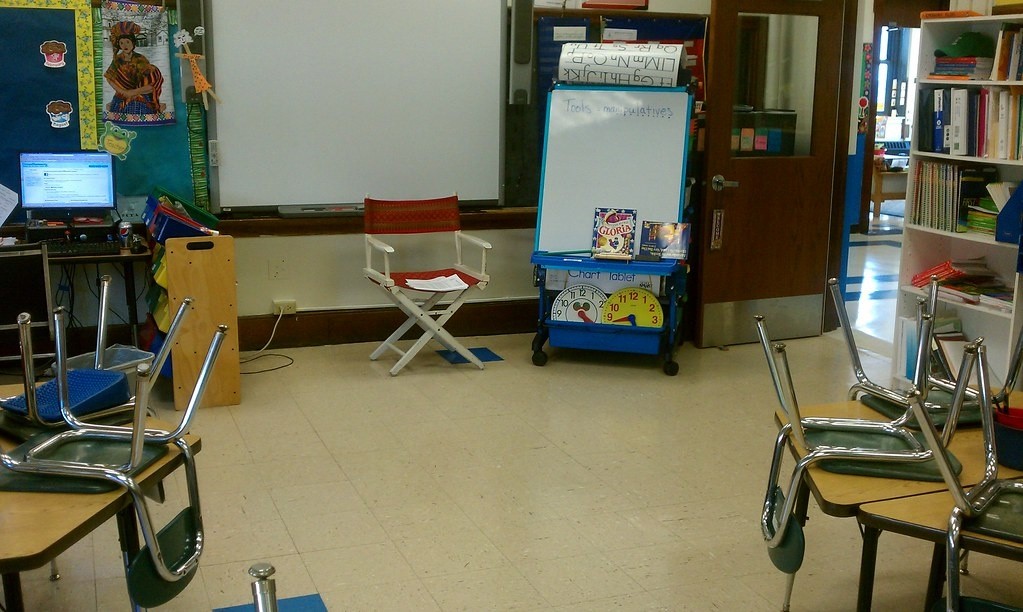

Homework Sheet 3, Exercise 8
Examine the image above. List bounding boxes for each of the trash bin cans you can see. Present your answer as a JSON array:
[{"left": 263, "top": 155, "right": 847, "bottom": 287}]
[{"left": 51, "top": 343, "right": 156, "bottom": 398}]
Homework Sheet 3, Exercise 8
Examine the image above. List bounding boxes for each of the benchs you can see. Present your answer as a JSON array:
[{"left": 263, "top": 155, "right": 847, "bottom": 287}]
[{"left": 871, "top": 165, "right": 909, "bottom": 217}]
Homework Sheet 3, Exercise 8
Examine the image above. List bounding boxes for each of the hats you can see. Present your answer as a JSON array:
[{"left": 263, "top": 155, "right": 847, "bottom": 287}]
[{"left": 934, "top": 31, "right": 994, "bottom": 58}]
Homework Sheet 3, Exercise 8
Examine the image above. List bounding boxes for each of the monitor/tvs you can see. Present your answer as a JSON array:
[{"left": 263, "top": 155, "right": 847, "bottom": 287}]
[{"left": 16, "top": 149, "right": 118, "bottom": 221}]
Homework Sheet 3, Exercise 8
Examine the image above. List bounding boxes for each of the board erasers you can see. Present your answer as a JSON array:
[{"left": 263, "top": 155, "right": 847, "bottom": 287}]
[
  {"left": 635, "top": 255, "right": 661, "bottom": 262},
  {"left": 594, "top": 254, "right": 632, "bottom": 264}
]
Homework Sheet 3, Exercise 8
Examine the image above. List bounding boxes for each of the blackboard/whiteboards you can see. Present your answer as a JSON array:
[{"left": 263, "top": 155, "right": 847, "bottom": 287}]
[
  {"left": 205, "top": 0, "right": 507, "bottom": 215},
  {"left": 506, "top": 11, "right": 770, "bottom": 215},
  {"left": 533, "top": 84, "right": 693, "bottom": 273},
  {"left": 0, "top": 1, "right": 95, "bottom": 228}
]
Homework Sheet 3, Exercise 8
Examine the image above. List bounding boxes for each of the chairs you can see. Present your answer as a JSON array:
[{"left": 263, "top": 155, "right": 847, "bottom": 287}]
[
  {"left": 890, "top": 157, "right": 909, "bottom": 170},
  {"left": 753, "top": 272, "right": 1023, "bottom": 612},
  {"left": 362, "top": 196, "right": 492, "bottom": 374},
  {"left": 0, "top": 274, "right": 192, "bottom": 504},
  {"left": 0, "top": 307, "right": 228, "bottom": 608}
]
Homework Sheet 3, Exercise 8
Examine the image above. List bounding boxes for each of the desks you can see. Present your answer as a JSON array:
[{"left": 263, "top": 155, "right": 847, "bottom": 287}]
[
  {"left": 880, "top": 154, "right": 912, "bottom": 159},
  {"left": 47, "top": 233, "right": 153, "bottom": 353},
  {"left": 775, "top": 386, "right": 1023, "bottom": 612},
  {"left": 0, "top": 378, "right": 202, "bottom": 612}
]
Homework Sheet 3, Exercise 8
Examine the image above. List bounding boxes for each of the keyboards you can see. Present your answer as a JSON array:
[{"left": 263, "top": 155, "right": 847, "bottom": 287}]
[{"left": 38, "top": 237, "right": 121, "bottom": 258}]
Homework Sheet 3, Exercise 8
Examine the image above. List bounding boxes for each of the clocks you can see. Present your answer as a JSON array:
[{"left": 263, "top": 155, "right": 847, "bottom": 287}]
[{"left": 550, "top": 283, "right": 664, "bottom": 329}]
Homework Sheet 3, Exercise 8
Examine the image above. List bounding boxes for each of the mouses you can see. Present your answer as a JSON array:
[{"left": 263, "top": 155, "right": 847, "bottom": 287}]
[{"left": 131, "top": 244, "right": 148, "bottom": 254}]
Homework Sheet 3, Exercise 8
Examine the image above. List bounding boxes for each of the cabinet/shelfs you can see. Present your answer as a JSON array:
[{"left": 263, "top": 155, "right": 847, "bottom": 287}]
[{"left": 891, "top": 13, "right": 1023, "bottom": 397}]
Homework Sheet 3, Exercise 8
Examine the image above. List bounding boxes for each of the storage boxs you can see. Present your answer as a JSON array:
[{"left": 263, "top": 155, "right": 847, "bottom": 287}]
[
  {"left": 138, "top": 185, "right": 220, "bottom": 377},
  {"left": 530, "top": 248, "right": 680, "bottom": 376}
]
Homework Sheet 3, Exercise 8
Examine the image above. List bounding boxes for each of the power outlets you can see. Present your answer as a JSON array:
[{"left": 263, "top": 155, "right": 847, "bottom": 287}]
[{"left": 273, "top": 299, "right": 298, "bottom": 315}]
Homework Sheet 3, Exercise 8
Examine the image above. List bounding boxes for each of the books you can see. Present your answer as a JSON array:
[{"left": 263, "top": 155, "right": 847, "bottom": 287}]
[
  {"left": 918, "top": 85, "right": 1023, "bottom": 161},
  {"left": 910, "top": 160, "right": 1023, "bottom": 243},
  {"left": 927, "top": 22, "right": 1023, "bottom": 81},
  {"left": 897, "top": 316, "right": 1004, "bottom": 396},
  {"left": 910, "top": 256, "right": 1014, "bottom": 314}
]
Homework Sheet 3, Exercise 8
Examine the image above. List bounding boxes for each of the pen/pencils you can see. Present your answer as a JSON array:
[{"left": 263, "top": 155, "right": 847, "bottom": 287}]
[{"left": 990, "top": 385, "right": 1010, "bottom": 414}]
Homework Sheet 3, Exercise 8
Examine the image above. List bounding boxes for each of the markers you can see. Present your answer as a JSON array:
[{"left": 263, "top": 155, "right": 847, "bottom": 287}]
[
  {"left": 328, "top": 206, "right": 357, "bottom": 211},
  {"left": 538, "top": 248, "right": 602, "bottom": 256},
  {"left": 301, "top": 207, "right": 327, "bottom": 211}
]
